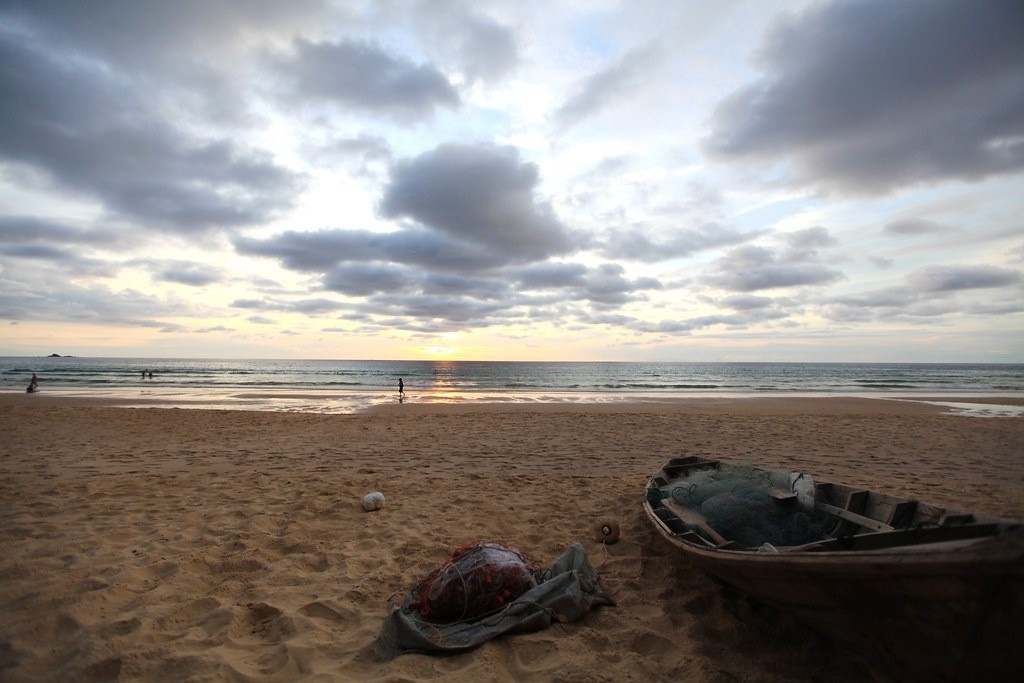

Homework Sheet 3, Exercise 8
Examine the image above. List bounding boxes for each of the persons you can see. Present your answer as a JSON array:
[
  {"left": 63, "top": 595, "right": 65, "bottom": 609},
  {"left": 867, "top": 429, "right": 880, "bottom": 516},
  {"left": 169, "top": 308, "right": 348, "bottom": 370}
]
[
  {"left": 433, "top": 370, "right": 438, "bottom": 374},
  {"left": 398, "top": 378, "right": 405, "bottom": 396},
  {"left": 31, "top": 373, "right": 38, "bottom": 386}
]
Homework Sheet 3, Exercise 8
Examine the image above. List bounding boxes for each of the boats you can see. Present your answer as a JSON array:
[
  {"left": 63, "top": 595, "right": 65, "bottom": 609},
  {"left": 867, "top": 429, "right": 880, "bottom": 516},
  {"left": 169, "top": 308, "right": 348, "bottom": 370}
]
[{"left": 643, "top": 456, "right": 1023, "bottom": 613}]
